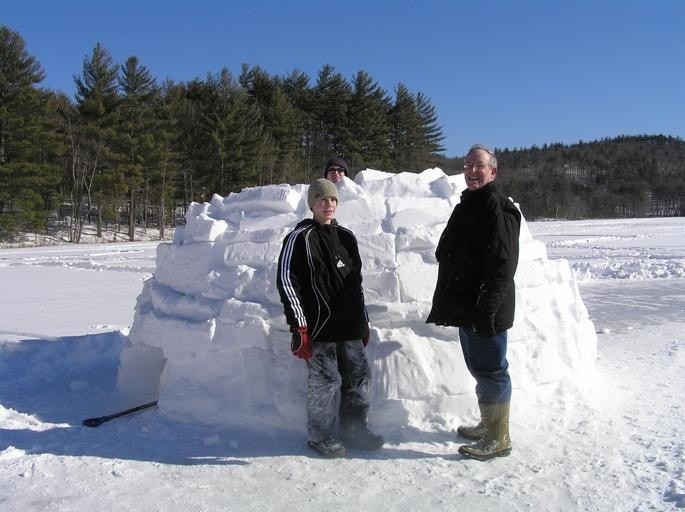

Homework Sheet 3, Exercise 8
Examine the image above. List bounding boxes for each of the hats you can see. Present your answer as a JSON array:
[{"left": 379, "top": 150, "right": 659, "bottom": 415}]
[
  {"left": 324, "top": 157, "right": 348, "bottom": 176},
  {"left": 307, "top": 178, "right": 339, "bottom": 211}
]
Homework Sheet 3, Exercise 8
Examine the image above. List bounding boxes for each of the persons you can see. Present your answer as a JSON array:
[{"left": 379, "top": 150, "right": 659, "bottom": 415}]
[
  {"left": 422, "top": 143, "right": 523, "bottom": 461},
  {"left": 274, "top": 177, "right": 386, "bottom": 459},
  {"left": 325, "top": 156, "right": 349, "bottom": 184}
]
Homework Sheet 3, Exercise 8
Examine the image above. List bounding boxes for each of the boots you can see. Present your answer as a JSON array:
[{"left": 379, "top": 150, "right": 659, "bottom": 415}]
[
  {"left": 457, "top": 401, "right": 511, "bottom": 461},
  {"left": 309, "top": 420, "right": 383, "bottom": 458}
]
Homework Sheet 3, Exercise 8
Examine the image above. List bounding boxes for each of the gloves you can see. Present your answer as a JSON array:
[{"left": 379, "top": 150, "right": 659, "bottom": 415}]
[
  {"left": 361, "top": 322, "right": 370, "bottom": 348},
  {"left": 290, "top": 328, "right": 315, "bottom": 359}
]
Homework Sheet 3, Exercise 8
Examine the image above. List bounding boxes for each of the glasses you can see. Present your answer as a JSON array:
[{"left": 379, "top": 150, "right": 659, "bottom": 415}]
[
  {"left": 328, "top": 168, "right": 344, "bottom": 172},
  {"left": 464, "top": 162, "right": 488, "bottom": 169}
]
